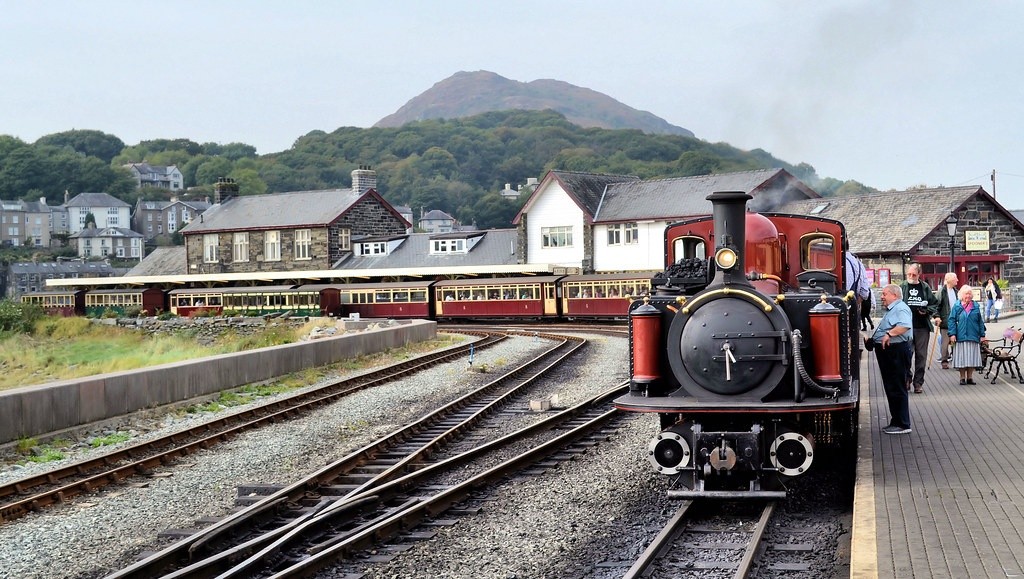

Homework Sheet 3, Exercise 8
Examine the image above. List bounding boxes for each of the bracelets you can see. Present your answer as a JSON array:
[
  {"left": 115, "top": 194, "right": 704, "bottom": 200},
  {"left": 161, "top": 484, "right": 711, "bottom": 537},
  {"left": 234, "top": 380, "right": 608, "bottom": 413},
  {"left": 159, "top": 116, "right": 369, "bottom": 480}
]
[{"left": 885, "top": 331, "right": 892, "bottom": 338}]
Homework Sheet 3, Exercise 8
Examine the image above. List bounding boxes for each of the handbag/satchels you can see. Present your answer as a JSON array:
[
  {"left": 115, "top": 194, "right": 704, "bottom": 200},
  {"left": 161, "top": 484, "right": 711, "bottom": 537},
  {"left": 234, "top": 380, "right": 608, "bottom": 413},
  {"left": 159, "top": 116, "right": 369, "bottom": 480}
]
[{"left": 995, "top": 299, "right": 1003, "bottom": 310}]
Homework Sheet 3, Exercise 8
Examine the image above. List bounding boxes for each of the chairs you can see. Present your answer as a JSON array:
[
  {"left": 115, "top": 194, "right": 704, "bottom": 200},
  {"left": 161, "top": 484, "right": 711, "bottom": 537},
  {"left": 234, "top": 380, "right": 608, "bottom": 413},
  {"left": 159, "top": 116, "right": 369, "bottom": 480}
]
[{"left": 981, "top": 326, "right": 1024, "bottom": 384}]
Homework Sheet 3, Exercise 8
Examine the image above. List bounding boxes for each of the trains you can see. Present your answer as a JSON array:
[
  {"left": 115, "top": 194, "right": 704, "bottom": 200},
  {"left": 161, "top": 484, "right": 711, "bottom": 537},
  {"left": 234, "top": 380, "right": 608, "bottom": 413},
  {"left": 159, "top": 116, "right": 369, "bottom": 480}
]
[{"left": 20, "top": 191, "right": 870, "bottom": 499}]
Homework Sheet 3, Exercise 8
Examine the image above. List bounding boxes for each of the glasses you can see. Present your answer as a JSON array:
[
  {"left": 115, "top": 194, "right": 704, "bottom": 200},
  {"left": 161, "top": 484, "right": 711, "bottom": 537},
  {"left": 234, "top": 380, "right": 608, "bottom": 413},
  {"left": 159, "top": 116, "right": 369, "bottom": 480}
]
[{"left": 908, "top": 273, "right": 919, "bottom": 277}]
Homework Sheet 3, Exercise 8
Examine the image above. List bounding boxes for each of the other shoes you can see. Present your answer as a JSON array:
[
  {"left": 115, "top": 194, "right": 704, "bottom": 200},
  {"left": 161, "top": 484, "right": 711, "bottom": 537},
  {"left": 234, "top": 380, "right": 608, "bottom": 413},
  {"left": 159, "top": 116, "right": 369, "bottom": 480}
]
[
  {"left": 936, "top": 356, "right": 952, "bottom": 362},
  {"left": 960, "top": 379, "right": 966, "bottom": 385},
  {"left": 992, "top": 318, "right": 997, "bottom": 323},
  {"left": 882, "top": 424, "right": 912, "bottom": 434},
  {"left": 967, "top": 379, "right": 976, "bottom": 385},
  {"left": 914, "top": 383, "right": 923, "bottom": 393},
  {"left": 985, "top": 318, "right": 990, "bottom": 323},
  {"left": 906, "top": 381, "right": 910, "bottom": 390},
  {"left": 941, "top": 362, "right": 949, "bottom": 369}
]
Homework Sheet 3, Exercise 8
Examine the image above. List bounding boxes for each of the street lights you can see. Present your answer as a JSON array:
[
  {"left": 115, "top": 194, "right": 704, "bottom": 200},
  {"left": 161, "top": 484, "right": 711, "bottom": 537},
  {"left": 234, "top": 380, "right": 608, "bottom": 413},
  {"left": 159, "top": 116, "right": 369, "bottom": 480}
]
[{"left": 945, "top": 210, "right": 959, "bottom": 273}]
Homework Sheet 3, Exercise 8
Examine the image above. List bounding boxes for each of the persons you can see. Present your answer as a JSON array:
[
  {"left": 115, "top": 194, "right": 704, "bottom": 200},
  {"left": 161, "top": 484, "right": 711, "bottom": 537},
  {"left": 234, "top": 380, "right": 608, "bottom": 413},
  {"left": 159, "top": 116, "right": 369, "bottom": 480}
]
[
  {"left": 871, "top": 284, "right": 914, "bottom": 435},
  {"left": 861, "top": 287, "right": 874, "bottom": 332},
  {"left": 845, "top": 239, "right": 869, "bottom": 311},
  {"left": 573, "top": 286, "right": 649, "bottom": 298},
  {"left": 899, "top": 262, "right": 1002, "bottom": 393},
  {"left": 180, "top": 298, "right": 215, "bottom": 306},
  {"left": 446, "top": 290, "right": 530, "bottom": 301}
]
